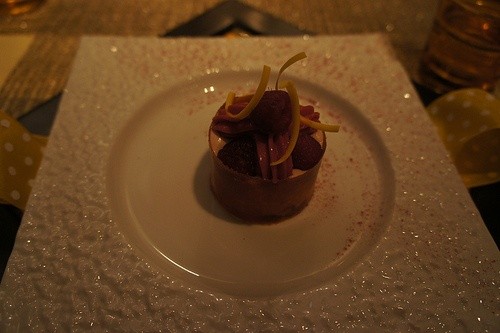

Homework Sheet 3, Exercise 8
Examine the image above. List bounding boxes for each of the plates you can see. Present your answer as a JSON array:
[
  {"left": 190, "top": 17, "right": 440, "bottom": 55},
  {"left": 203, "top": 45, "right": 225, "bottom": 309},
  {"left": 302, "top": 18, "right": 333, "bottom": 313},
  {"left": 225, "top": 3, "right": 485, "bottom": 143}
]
[{"left": 0, "top": 31, "right": 500, "bottom": 332}]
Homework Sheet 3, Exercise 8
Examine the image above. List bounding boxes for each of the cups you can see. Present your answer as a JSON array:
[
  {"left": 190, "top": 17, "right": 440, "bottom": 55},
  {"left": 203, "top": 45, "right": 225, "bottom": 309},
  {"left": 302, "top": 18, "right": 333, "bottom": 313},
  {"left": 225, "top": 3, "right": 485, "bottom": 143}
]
[
  {"left": 208, "top": 98, "right": 326, "bottom": 221},
  {"left": 415, "top": 0, "right": 500, "bottom": 96}
]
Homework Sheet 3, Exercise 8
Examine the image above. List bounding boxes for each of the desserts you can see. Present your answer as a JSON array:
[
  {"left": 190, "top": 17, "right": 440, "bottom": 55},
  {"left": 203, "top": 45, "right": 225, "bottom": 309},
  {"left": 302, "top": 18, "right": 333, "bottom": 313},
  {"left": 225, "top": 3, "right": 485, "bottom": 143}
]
[{"left": 208, "top": 52, "right": 340, "bottom": 224}]
